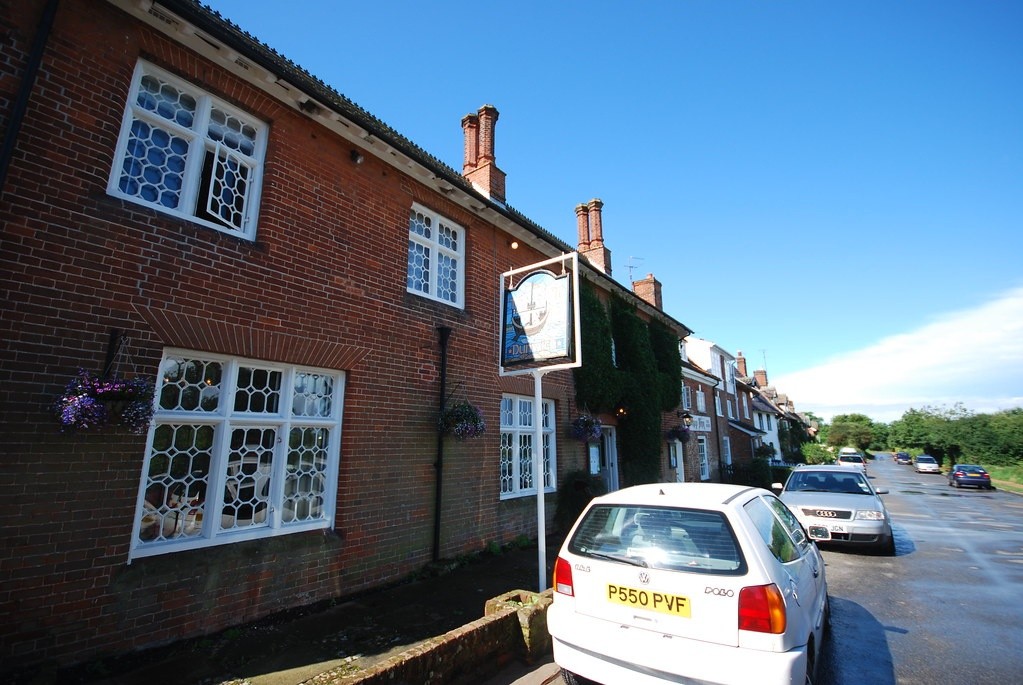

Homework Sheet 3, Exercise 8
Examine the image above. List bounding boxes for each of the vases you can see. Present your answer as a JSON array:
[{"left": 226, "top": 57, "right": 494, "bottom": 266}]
[
  {"left": 97, "top": 391, "right": 128, "bottom": 416},
  {"left": 680, "top": 438, "right": 689, "bottom": 444}
]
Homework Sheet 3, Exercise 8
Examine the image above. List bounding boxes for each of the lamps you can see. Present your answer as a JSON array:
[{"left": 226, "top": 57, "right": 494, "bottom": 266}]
[
  {"left": 351, "top": 150, "right": 365, "bottom": 165},
  {"left": 677, "top": 410, "right": 693, "bottom": 428}
]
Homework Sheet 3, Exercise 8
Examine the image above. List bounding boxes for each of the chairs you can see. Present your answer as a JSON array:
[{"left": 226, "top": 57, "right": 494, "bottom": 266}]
[
  {"left": 592, "top": 534, "right": 623, "bottom": 552},
  {"left": 807, "top": 476, "right": 820, "bottom": 487},
  {"left": 843, "top": 478, "right": 855, "bottom": 489}
]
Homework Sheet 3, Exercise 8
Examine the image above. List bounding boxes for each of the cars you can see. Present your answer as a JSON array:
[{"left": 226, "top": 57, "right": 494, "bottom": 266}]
[
  {"left": 894, "top": 453, "right": 897, "bottom": 461},
  {"left": 897, "top": 452, "right": 912, "bottom": 464},
  {"left": 948, "top": 464, "right": 991, "bottom": 489},
  {"left": 838, "top": 453, "right": 867, "bottom": 476},
  {"left": 547, "top": 482, "right": 827, "bottom": 685},
  {"left": 771, "top": 463, "right": 895, "bottom": 552},
  {"left": 913, "top": 455, "right": 939, "bottom": 473}
]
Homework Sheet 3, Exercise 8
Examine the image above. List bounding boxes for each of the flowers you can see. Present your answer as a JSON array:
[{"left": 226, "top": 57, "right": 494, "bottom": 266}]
[
  {"left": 675, "top": 425, "right": 690, "bottom": 439},
  {"left": 572, "top": 414, "right": 606, "bottom": 441},
  {"left": 436, "top": 399, "right": 488, "bottom": 440},
  {"left": 48, "top": 366, "right": 159, "bottom": 436}
]
